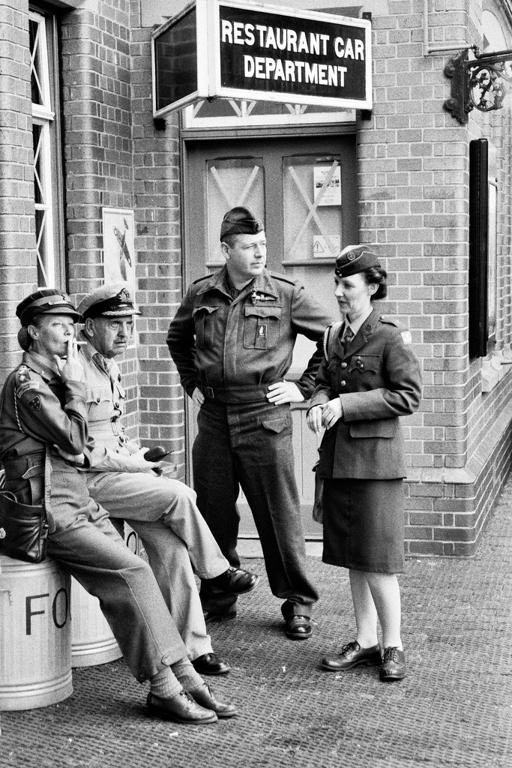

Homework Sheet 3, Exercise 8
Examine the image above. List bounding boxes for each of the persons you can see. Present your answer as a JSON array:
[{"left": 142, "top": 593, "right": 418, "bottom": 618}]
[
  {"left": 0, "top": 289, "right": 237, "bottom": 724},
  {"left": 63, "top": 286, "right": 257, "bottom": 675},
  {"left": 306, "top": 246, "right": 421, "bottom": 680},
  {"left": 165, "top": 206, "right": 336, "bottom": 639}
]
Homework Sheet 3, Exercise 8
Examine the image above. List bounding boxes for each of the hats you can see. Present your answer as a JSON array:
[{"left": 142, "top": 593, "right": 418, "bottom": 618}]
[
  {"left": 334, "top": 244, "right": 382, "bottom": 279},
  {"left": 220, "top": 205, "right": 265, "bottom": 244},
  {"left": 15, "top": 288, "right": 85, "bottom": 328},
  {"left": 75, "top": 280, "right": 143, "bottom": 324}
]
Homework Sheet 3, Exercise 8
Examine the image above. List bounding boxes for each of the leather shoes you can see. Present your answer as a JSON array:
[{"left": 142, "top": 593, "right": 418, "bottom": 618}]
[
  {"left": 379, "top": 645, "right": 407, "bottom": 682},
  {"left": 320, "top": 638, "right": 382, "bottom": 672},
  {"left": 284, "top": 614, "right": 314, "bottom": 639},
  {"left": 145, "top": 690, "right": 218, "bottom": 725},
  {"left": 202, "top": 603, "right": 238, "bottom": 626},
  {"left": 188, "top": 681, "right": 238, "bottom": 718},
  {"left": 200, "top": 565, "right": 260, "bottom": 596},
  {"left": 191, "top": 651, "right": 231, "bottom": 675}
]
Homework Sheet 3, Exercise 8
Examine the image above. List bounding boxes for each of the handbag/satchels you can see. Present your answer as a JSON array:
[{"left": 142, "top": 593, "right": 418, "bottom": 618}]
[{"left": 312, "top": 459, "right": 326, "bottom": 526}]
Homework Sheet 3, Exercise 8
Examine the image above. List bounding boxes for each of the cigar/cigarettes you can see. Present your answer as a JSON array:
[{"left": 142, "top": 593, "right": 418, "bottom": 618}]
[{"left": 67, "top": 340, "right": 87, "bottom": 346}]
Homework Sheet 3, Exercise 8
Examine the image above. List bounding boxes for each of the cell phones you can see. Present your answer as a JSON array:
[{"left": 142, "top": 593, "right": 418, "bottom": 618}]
[{"left": 143, "top": 446, "right": 174, "bottom": 462}]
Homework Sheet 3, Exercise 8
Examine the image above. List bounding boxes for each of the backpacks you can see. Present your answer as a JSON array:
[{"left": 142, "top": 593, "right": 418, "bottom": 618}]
[{"left": 0, "top": 486, "right": 55, "bottom": 565}]
